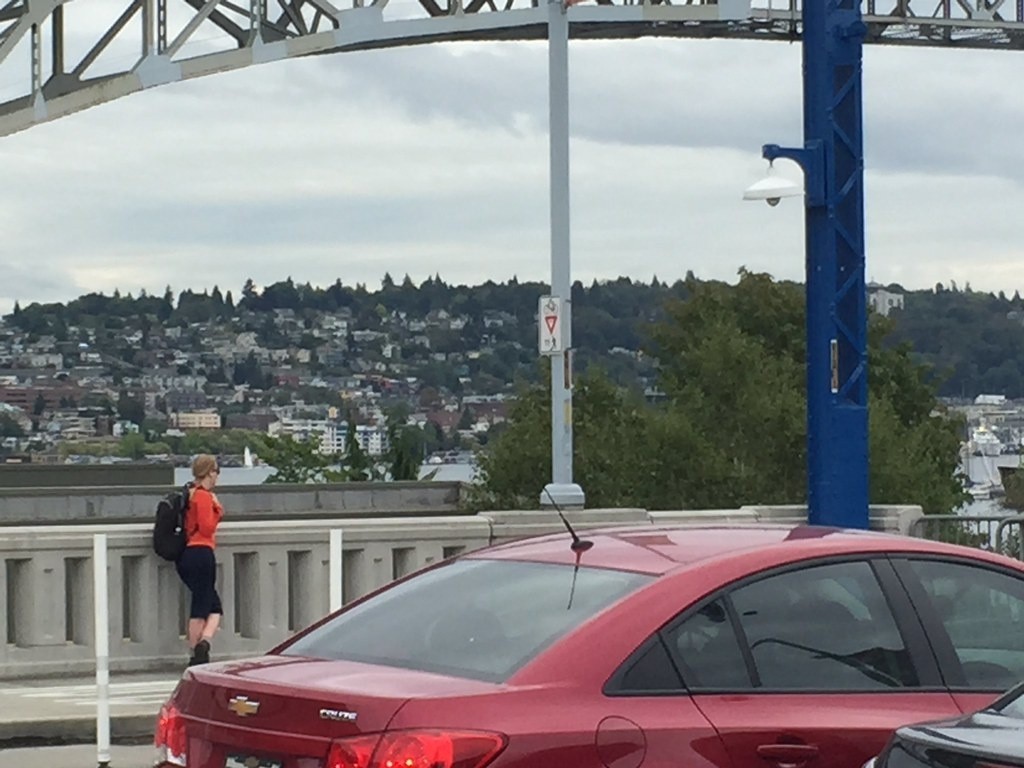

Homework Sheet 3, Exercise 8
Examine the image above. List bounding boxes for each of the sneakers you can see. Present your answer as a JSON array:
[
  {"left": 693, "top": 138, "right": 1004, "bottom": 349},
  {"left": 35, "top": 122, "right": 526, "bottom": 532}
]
[{"left": 187, "top": 639, "right": 212, "bottom": 666}]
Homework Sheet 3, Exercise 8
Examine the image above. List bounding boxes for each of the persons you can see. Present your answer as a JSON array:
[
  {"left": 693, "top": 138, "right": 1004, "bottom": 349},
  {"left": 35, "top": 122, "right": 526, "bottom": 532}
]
[{"left": 172, "top": 452, "right": 227, "bottom": 665}]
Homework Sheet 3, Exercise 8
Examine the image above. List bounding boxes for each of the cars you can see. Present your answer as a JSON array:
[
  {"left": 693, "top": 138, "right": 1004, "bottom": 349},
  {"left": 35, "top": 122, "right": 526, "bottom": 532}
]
[{"left": 152, "top": 520, "right": 1022, "bottom": 767}]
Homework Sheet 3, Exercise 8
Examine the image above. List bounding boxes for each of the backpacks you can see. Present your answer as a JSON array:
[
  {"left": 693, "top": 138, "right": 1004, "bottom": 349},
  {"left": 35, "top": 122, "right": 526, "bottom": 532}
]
[{"left": 152, "top": 483, "right": 200, "bottom": 560}]
